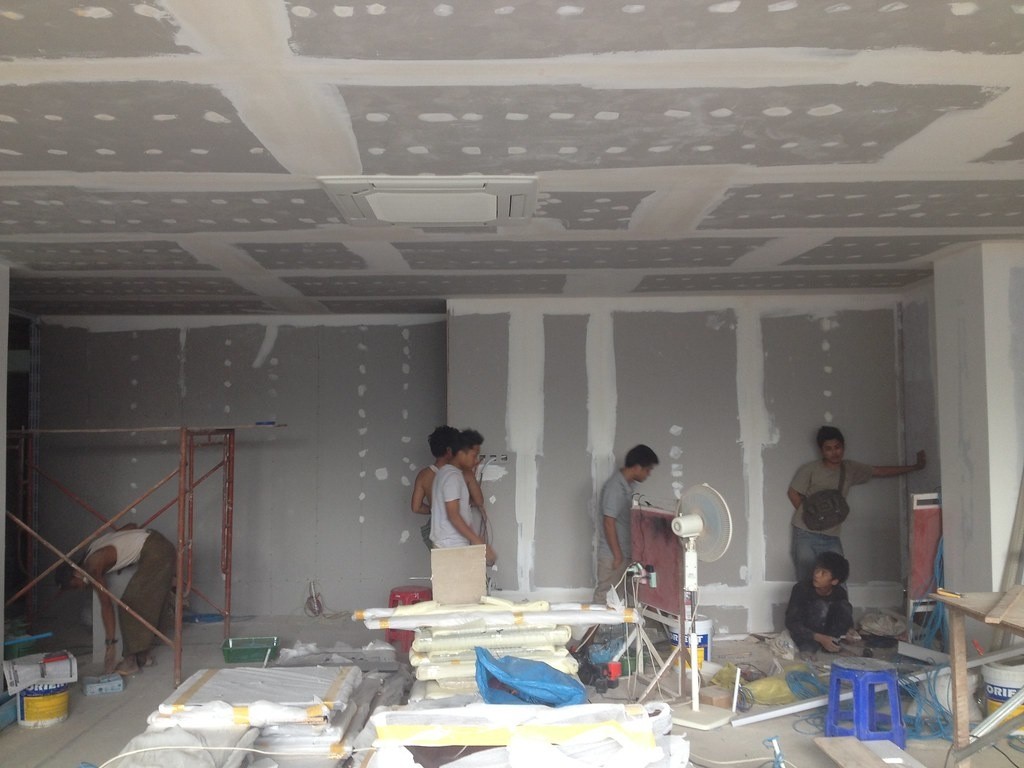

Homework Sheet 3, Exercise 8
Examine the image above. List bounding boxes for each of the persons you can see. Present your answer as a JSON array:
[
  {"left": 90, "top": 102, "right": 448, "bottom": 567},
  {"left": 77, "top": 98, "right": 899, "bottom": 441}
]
[
  {"left": 785, "top": 551, "right": 860, "bottom": 662},
  {"left": 788, "top": 427, "right": 926, "bottom": 640},
  {"left": 428, "top": 428, "right": 497, "bottom": 567},
  {"left": 578, "top": 445, "right": 669, "bottom": 656},
  {"left": 55, "top": 523, "right": 178, "bottom": 676},
  {"left": 411, "top": 426, "right": 483, "bottom": 548}
]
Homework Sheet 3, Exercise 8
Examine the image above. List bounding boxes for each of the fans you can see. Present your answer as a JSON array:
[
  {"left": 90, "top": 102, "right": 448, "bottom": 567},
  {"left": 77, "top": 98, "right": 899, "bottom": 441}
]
[{"left": 670, "top": 482, "right": 737, "bottom": 730}]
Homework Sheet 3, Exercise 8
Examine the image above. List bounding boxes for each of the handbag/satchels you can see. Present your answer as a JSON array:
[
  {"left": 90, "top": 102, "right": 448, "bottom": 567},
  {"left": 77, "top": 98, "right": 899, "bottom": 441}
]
[
  {"left": 802, "top": 462, "right": 849, "bottom": 532},
  {"left": 474, "top": 645, "right": 587, "bottom": 707}
]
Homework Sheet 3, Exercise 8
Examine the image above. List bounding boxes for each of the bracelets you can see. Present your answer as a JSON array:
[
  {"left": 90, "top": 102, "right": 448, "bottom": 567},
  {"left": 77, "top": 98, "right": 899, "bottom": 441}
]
[{"left": 105, "top": 640, "right": 118, "bottom": 644}]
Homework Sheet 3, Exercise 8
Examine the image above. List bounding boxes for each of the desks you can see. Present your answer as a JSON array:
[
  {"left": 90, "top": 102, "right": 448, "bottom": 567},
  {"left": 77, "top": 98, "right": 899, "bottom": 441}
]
[{"left": 927, "top": 590, "right": 1024, "bottom": 768}]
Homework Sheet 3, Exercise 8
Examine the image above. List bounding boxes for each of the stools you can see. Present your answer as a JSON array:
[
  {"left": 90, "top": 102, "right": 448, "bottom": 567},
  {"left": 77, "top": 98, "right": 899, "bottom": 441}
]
[
  {"left": 387, "top": 585, "right": 434, "bottom": 651},
  {"left": 826, "top": 656, "right": 907, "bottom": 750}
]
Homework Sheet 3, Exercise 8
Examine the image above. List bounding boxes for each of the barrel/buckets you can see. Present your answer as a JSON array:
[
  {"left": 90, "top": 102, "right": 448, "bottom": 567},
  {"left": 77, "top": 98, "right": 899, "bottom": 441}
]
[
  {"left": 981, "top": 649, "right": 1024, "bottom": 737},
  {"left": 11, "top": 652, "right": 69, "bottom": 728},
  {"left": 670, "top": 613, "right": 711, "bottom": 673}
]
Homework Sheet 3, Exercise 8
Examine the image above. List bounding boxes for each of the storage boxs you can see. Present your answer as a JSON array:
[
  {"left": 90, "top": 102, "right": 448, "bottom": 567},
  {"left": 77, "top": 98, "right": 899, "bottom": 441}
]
[
  {"left": 219, "top": 637, "right": 280, "bottom": 664},
  {"left": 700, "top": 686, "right": 732, "bottom": 708},
  {"left": 83, "top": 672, "right": 124, "bottom": 696},
  {"left": 620, "top": 649, "right": 648, "bottom": 675}
]
